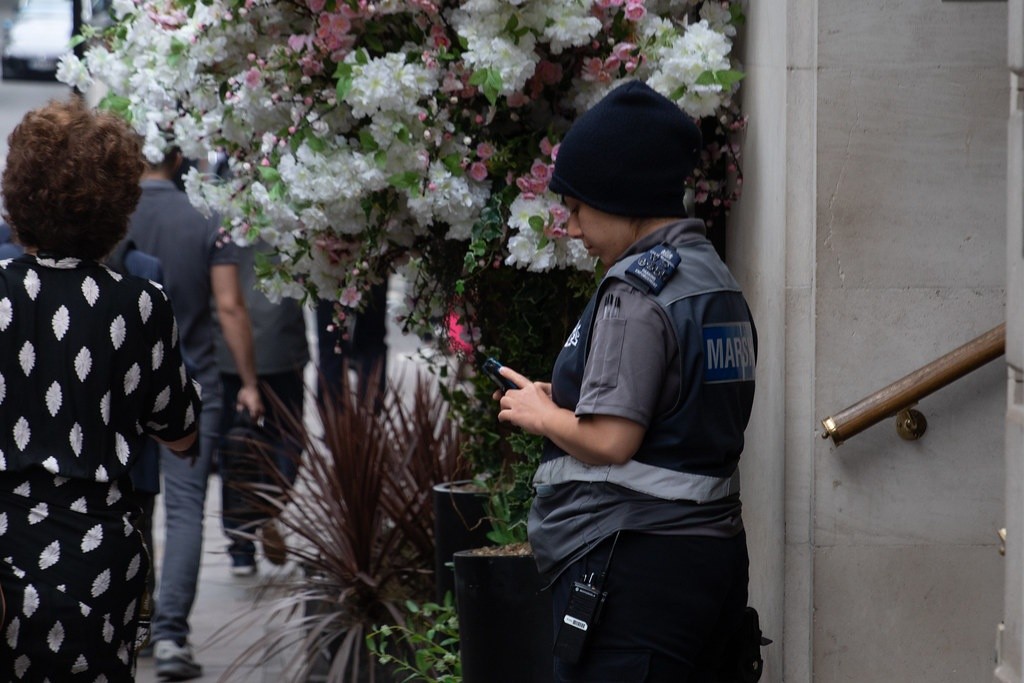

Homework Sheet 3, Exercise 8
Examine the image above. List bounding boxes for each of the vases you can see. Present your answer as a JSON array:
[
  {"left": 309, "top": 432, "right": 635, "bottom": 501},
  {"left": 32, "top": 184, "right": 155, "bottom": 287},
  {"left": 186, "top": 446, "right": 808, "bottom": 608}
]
[
  {"left": 453, "top": 546, "right": 554, "bottom": 683},
  {"left": 430, "top": 478, "right": 527, "bottom": 591}
]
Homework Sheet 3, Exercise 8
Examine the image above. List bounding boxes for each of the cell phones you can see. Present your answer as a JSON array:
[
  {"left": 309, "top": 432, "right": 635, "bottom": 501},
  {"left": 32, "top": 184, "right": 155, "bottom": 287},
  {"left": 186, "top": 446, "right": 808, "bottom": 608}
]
[{"left": 483, "top": 358, "right": 519, "bottom": 394}]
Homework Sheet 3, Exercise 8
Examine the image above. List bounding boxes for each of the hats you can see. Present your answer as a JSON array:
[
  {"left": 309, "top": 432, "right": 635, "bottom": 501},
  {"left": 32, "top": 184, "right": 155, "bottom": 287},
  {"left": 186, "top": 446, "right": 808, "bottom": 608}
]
[{"left": 547, "top": 79, "right": 702, "bottom": 219}]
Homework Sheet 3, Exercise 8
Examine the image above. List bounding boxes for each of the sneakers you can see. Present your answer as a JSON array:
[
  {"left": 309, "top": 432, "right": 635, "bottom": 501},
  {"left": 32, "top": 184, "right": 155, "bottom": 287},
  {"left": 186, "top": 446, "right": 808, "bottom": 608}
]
[{"left": 152, "top": 640, "right": 203, "bottom": 677}]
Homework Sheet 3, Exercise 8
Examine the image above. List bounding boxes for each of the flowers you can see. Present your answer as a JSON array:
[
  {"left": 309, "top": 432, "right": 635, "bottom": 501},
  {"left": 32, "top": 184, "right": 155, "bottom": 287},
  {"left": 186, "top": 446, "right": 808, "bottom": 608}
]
[{"left": 55, "top": 0, "right": 744, "bottom": 540}]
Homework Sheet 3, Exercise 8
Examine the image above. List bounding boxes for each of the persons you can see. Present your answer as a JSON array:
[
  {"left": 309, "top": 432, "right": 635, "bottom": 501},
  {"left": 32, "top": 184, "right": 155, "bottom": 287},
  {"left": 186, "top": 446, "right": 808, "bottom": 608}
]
[
  {"left": 493, "top": 82, "right": 772, "bottom": 683},
  {"left": 313, "top": 276, "right": 391, "bottom": 435},
  {"left": 1, "top": 106, "right": 204, "bottom": 683},
  {"left": 211, "top": 78, "right": 313, "bottom": 576},
  {"left": 122, "top": 123, "right": 265, "bottom": 682}
]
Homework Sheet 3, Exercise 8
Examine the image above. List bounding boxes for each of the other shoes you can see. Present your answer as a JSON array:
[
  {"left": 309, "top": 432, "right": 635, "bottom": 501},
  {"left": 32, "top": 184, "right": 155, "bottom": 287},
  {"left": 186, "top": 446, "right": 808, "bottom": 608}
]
[
  {"left": 229, "top": 542, "right": 256, "bottom": 576},
  {"left": 262, "top": 518, "right": 288, "bottom": 567}
]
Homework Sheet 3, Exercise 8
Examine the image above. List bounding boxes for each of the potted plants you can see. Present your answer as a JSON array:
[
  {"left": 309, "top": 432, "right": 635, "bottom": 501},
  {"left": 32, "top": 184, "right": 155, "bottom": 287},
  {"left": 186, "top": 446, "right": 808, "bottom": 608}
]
[{"left": 189, "top": 353, "right": 468, "bottom": 683}]
